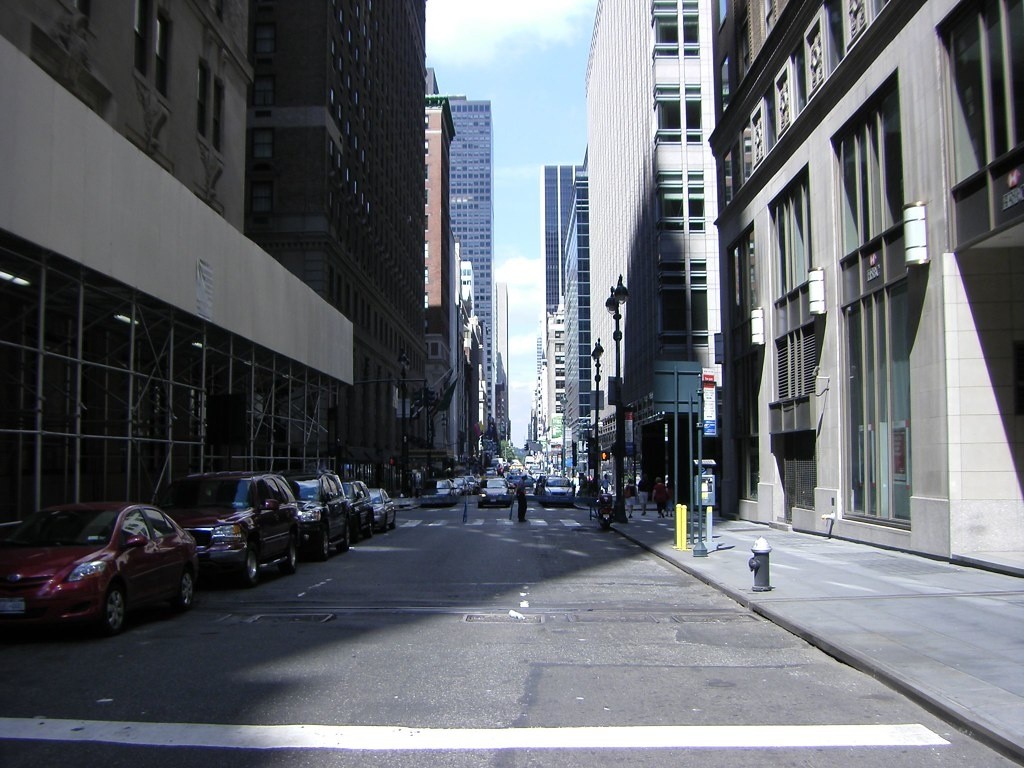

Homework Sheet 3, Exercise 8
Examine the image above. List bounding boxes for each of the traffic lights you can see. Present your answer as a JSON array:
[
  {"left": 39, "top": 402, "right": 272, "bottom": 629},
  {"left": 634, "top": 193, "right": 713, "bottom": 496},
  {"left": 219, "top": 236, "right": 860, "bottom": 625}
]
[{"left": 600, "top": 451, "right": 609, "bottom": 461}]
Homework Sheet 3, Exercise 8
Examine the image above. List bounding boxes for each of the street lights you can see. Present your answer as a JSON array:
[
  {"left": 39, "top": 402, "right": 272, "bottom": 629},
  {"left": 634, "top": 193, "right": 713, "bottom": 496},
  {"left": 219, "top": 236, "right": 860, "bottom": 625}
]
[
  {"left": 591, "top": 338, "right": 604, "bottom": 496},
  {"left": 606, "top": 274, "right": 630, "bottom": 523}
]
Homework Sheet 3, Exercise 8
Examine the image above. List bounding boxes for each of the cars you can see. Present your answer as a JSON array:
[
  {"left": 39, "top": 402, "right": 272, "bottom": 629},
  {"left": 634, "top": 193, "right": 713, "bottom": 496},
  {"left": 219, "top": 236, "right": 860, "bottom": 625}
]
[
  {"left": 419, "top": 478, "right": 458, "bottom": 507},
  {"left": 0, "top": 501, "right": 200, "bottom": 634},
  {"left": 539, "top": 476, "right": 575, "bottom": 506},
  {"left": 453, "top": 456, "right": 543, "bottom": 494},
  {"left": 476, "top": 478, "right": 513, "bottom": 507}
]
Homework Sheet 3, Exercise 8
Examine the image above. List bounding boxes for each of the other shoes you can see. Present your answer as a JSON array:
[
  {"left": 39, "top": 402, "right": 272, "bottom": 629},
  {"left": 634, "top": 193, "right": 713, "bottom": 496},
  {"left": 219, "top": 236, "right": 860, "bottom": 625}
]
[
  {"left": 629, "top": 514, "right": 632, "bottom": 518},
  {"left": 519, "top": 519, "right": 526, "bottom": 522},
  {"left": 657, "top": 513, "right": 661, "bottom": 518},
  {"left": 670, "top": 514, "right": 673, "bottom": 517},
  {"left": 662, "top": 513, "right": 665, "bottom": 518},
  {"left": 641, "top": 511, "right": 646, "bottom": 516}
]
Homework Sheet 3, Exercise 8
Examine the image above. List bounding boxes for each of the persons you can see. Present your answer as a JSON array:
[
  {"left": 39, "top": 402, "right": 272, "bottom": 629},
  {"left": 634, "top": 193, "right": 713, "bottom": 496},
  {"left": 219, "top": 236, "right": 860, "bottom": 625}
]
[
  {"left": 498, "top": 465, "right": 504, "bottom": 478},
  {"left": 540, "top": 478, "right": 545, "bottom": 495},
  {"left": 589, "top": 475, "right": 594, "bottom": 482},
  {"left": 517, "top": 474, "right": 528, "bottom": 522},
  {"left": 652, "top": 477, "right": 673, "bottom": 518},
  {"left": 638, "top": 474, "right": 650, "bottom": 515},
  {"left": 601, "top": 474, "right": 613, "bottom": 494},
  {"left": 517, "top": 470, "right": 523, "bottom": 475},
  {"left": 626, "top": 479, "right": 638, "bottom": 518}
]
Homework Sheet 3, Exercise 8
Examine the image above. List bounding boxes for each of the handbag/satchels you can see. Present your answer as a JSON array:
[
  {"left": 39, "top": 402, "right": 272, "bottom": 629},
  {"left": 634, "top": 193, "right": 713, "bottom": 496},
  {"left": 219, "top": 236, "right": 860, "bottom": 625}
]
[{"left": 624, "top": 485, "right": 630, "bottom": 498}]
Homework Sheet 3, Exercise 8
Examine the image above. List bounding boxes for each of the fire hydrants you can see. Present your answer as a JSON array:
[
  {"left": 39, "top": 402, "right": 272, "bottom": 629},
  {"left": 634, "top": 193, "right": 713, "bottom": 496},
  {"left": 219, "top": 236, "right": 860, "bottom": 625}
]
[{"left": 749, "top": 535, "right": 772, "bottom": 591}]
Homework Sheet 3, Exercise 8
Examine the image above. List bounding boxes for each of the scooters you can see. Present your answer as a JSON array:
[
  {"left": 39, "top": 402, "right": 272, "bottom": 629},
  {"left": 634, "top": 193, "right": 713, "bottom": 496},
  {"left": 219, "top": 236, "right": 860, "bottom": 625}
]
[{"left": 594, "top": 493, "right": 615, "bottom": 528}]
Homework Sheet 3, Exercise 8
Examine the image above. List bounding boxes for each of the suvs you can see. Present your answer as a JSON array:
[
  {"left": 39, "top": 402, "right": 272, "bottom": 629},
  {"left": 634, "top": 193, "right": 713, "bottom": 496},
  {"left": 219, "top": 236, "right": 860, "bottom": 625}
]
[
  {"left": 274, "top": 468, "right": 396, "bottom": 561},
  {"left": 155, "top": 471, "right": 301, "bottom": 588}
]
